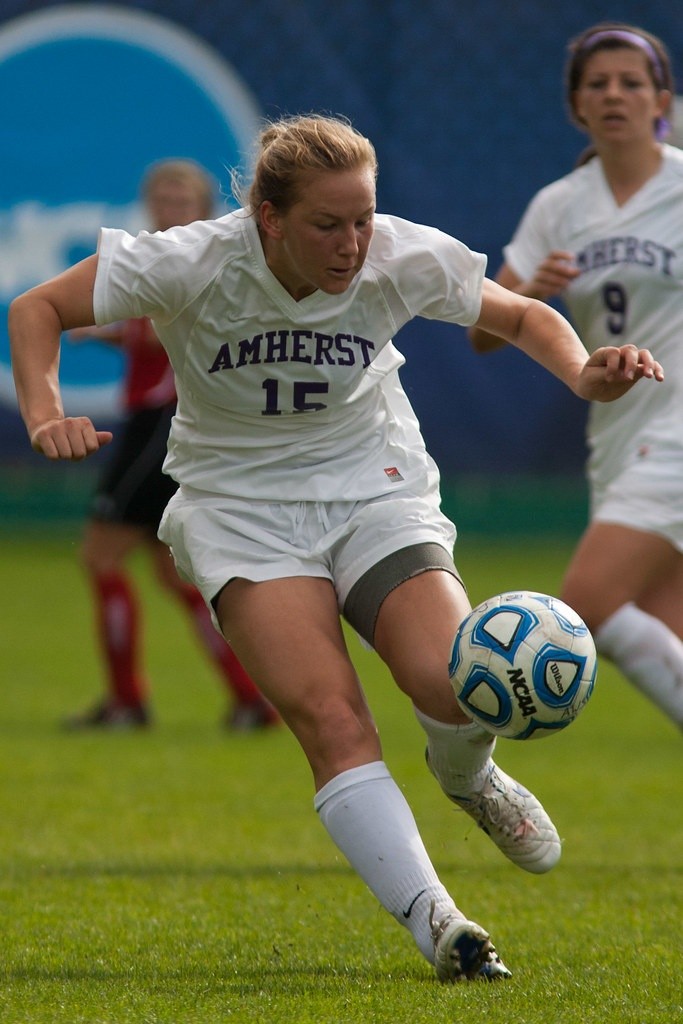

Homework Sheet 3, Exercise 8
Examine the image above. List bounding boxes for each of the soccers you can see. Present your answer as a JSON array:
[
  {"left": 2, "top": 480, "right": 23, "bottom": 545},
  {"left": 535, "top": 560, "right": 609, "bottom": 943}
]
[{"left": 447, "top": 590, "right": 598, "bottom": 742}]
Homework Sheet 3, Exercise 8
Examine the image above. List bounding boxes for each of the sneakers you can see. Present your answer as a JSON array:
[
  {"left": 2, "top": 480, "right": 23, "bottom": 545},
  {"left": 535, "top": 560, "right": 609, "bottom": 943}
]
[
  {"left": 427, "top": 897, "right": 512, "bottom": 980},
  {"left": 424, "top": 745, "right": 563, "bottom": 875}
]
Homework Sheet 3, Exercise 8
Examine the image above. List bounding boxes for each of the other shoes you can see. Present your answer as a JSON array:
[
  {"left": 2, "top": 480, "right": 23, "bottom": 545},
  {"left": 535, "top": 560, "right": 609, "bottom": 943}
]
[
  {"left": 60, "top": 701, "right": 142, "bottom": 732},
  {"left": 223, "top": 707, "right": 267, "bottom": 730}
]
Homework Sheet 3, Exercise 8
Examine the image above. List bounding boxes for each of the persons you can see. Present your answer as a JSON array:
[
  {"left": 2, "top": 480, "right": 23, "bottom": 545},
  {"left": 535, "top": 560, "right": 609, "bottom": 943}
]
[
  {"left": 64, "top": 160, "right": 284, "bottom": 731},
  {"left": 469, "top": 24, "right": 683, "bottom": 733},
  {"left": 8, "top": 114, "right": 664, "bottom": 986}
]
